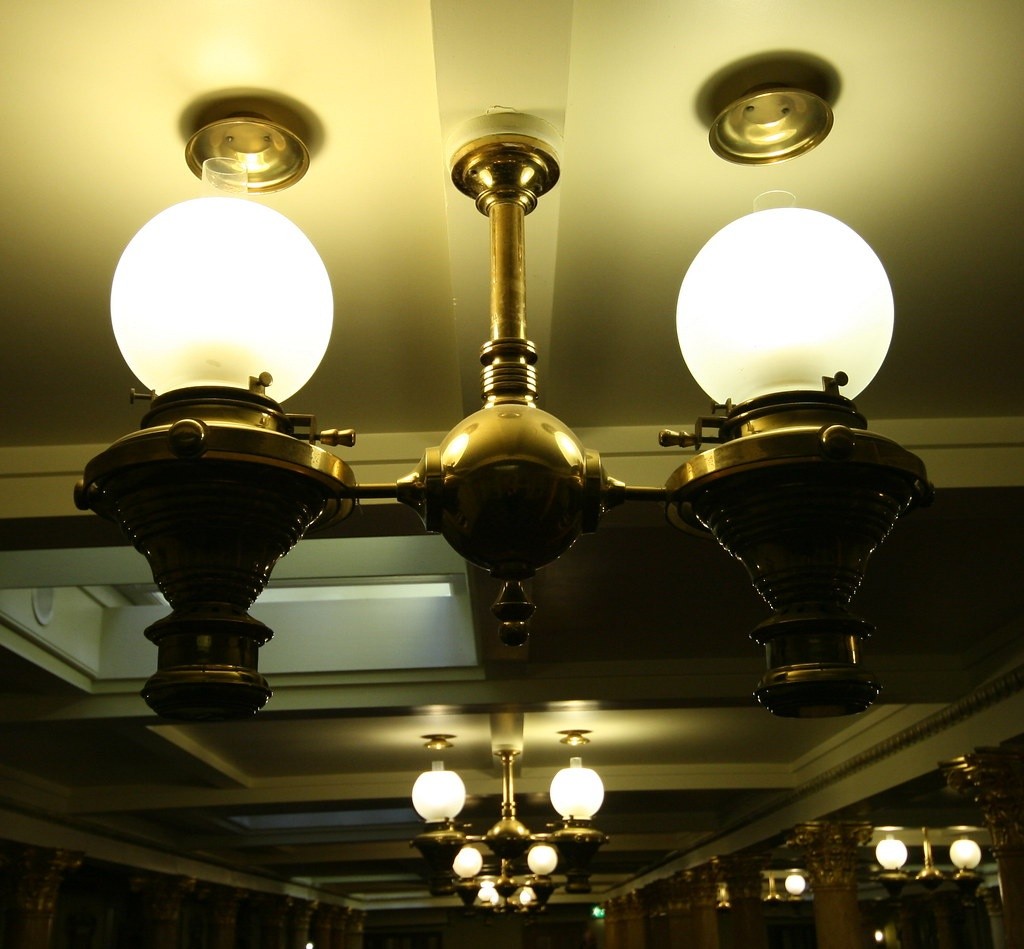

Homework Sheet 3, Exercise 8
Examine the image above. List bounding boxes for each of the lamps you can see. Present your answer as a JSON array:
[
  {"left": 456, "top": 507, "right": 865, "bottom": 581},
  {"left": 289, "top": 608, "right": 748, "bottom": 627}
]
[
  {"left": 549, "top": 757, "right": 610, "bottom": 868},
  {"left": 659, "top": 190, "right": 936, "bottom": 716},
  {"left": 409, "top": 761, "right": 473, "bottom": 879},
  {"left": 876, "top": 840, "right": 985, "bottom": 902},
  {"left": 73, "top": 158, "right": 357, "bottom": 721},
  {"left": 786, "top": 874, "right": 805, "bottom": 903},
  {"left": 449, "top": 842, "right": 558, "bottom": 922}
]
